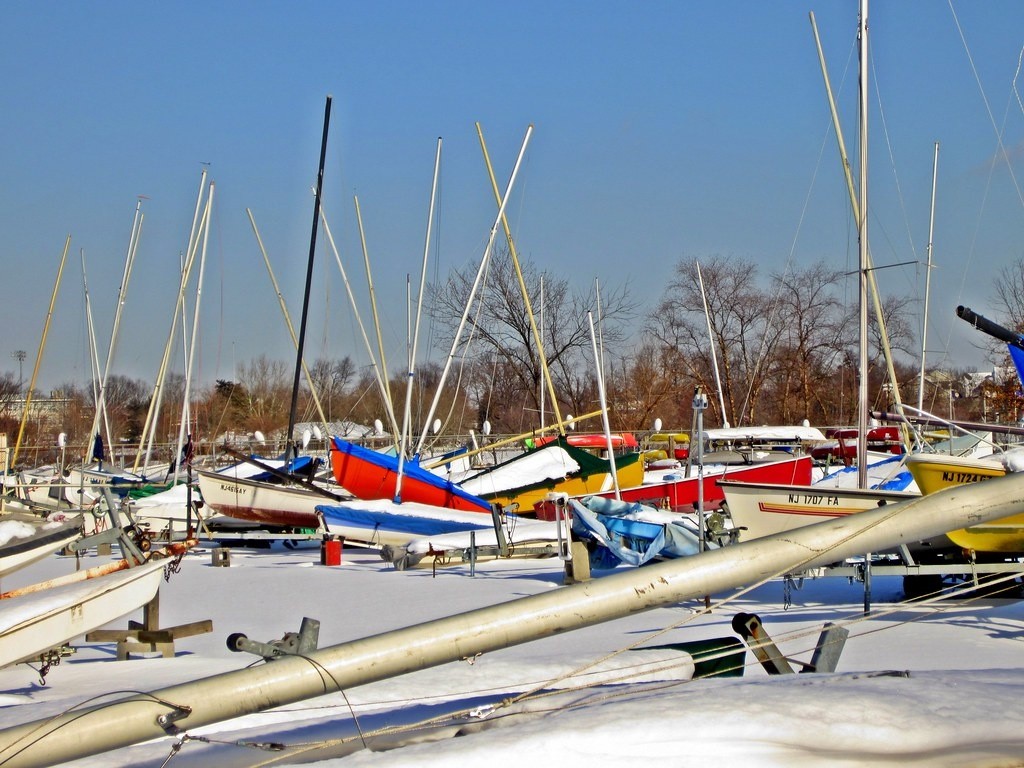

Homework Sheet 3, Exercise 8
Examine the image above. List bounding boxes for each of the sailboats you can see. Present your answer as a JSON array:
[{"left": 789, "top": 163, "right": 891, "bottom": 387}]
[{"left": 0, "top": 1, "right": 1024, "bottom": 671}]
